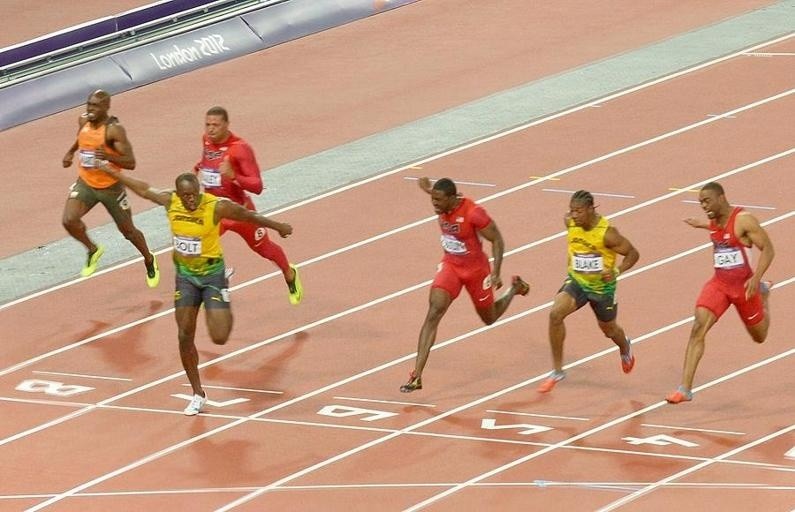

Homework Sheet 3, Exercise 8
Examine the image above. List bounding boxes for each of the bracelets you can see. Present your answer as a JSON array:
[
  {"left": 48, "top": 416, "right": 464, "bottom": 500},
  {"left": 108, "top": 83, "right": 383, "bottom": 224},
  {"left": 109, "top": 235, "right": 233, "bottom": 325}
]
[{"left": 610, "top": 269, "right": 621, "bottom": 278}]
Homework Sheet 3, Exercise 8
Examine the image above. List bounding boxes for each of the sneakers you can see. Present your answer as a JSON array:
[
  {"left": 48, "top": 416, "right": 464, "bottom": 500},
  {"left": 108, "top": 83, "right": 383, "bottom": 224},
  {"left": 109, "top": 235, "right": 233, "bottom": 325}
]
[
  {"left": 145, "top": 251, "right": 160, "bottom": 288},
  {"left": 82, "top": 244, "right": 104, "bottom": 276},
  {"left": 286, "top": 266, "right": 302, "bottom": 305},
  {"left": 538, "top": 370, "right": 566, "bottom": 393},
  {"left": 185, "top": 392, "right": 207, "bottom": 416},
  {"left": 511, "top": 274, "right": 529, "bottom": 296},
  {"left": 621, "top": 339, "right": 634, "bottom": 373},
  {"left": 400, "top": 374, "right": 422, "bottom": 393},
  {"left": 761, "top": 279, "right": 772, "bottom": 293},
  {"left": 664, "top": 387, "right": 692, "bottom": 404}
]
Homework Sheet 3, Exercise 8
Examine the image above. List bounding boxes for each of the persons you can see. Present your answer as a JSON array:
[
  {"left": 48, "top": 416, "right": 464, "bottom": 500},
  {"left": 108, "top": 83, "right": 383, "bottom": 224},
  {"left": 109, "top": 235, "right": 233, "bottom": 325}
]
[
  {"left": 92, "top": 156, "right": 293, "bottom": 417},
  {"left": 60, "top": 88, "right": 164, "bottom": 289},
  {"left": 397, "top": 176, "right": 530, "bottom": 396},
  {"left": 665, "top": 181, "right": 773, "bottom": 403},
  {"left": 536, "top": 189, "right": 641, "bottom": 394},
  {"left": 193, "top": 106, "right": 303, "bottom": 305}
]
